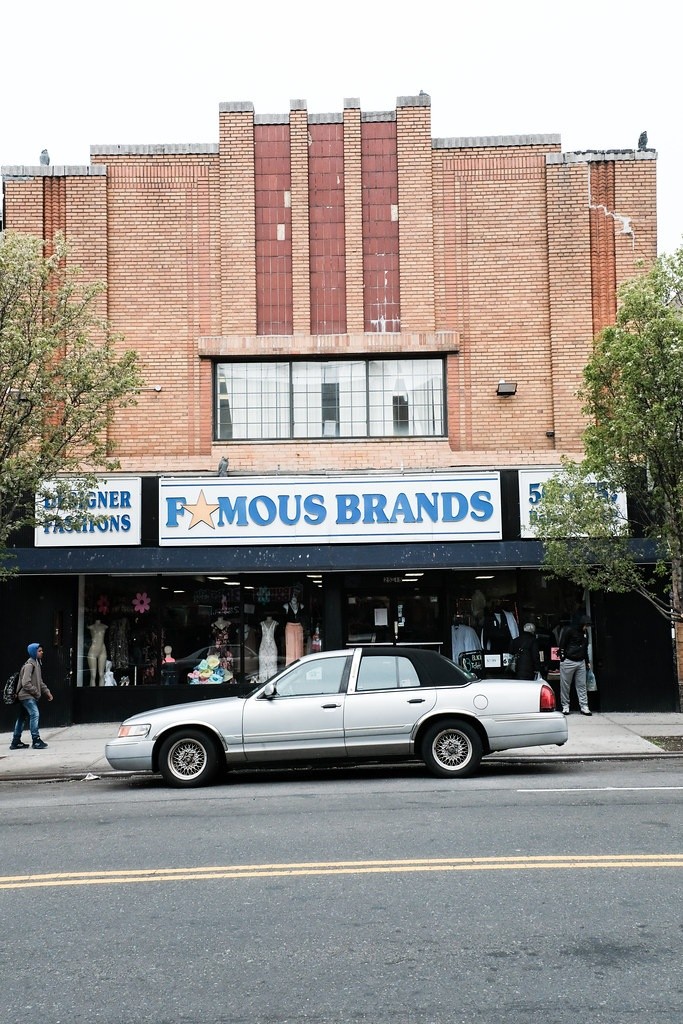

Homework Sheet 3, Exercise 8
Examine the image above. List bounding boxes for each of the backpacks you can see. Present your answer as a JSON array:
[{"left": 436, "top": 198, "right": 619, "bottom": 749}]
[{"left": 2, "top": 662, "right": 35, "bottom": 704}]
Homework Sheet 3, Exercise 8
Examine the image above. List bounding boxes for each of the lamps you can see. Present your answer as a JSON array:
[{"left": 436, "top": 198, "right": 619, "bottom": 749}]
[{"left": 495, "top": 383, "right": 517, "bottom": 397}]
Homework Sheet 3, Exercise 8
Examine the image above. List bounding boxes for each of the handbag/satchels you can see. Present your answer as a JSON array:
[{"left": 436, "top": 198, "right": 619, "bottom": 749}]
[
  {"left": 510, "top": 654, "right": 520, "bottom": 672},
  {"left": 586, "top": 668, "right": 597, "bottom": 691}
]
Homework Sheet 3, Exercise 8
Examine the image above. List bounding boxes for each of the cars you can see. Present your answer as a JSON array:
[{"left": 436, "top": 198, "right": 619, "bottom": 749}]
[
  {"left": 103, "top": 647, "right": 569, "bottom": 789},
  {"left": 163, "top": 645, "right": 293, "bottom": 684}
]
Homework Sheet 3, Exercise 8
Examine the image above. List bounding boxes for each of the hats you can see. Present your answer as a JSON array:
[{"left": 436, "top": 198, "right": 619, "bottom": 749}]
[
  {"left": 523, "top": 623, "right": 536, "bottom": 632},
  {"left": 580, "top": 614, "right": 593, "bottom": 624}
]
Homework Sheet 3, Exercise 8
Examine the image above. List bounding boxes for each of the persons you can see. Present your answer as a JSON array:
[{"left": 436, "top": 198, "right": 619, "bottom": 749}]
[
  {"left": 10, "top": 643, "right": 54, "bottom": 751},
  {"left": 511, "top": 623, "right": 557, "bottom": 681},
  {"left": 559, "top": 616, "right": 592, "bottom": 716},
  {"left": 87, "top": 620, "right": 108, "bottom": 686},
  {"left": 211, "top": 617, "right": 234, "bottom": 685},
  {"left": 259, "top": 617, "right": 279, "bottom": 683},
  {"left": 284, "top": 598, "right": 305, "bottom": 667}
]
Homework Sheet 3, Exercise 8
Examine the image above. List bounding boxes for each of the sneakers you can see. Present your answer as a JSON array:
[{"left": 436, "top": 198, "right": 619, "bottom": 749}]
[
  {"left": 32, "top": 736, "right": 48, "bottom": 749},
  {"left": 581, "top": 705, "right": 592, "bottom": 716},
  {"left": 9, "top": 739, "right": 29, "bottom": 750},
  {"left": 562, "top": 705, "right": 570, "bottom": 714}
]
